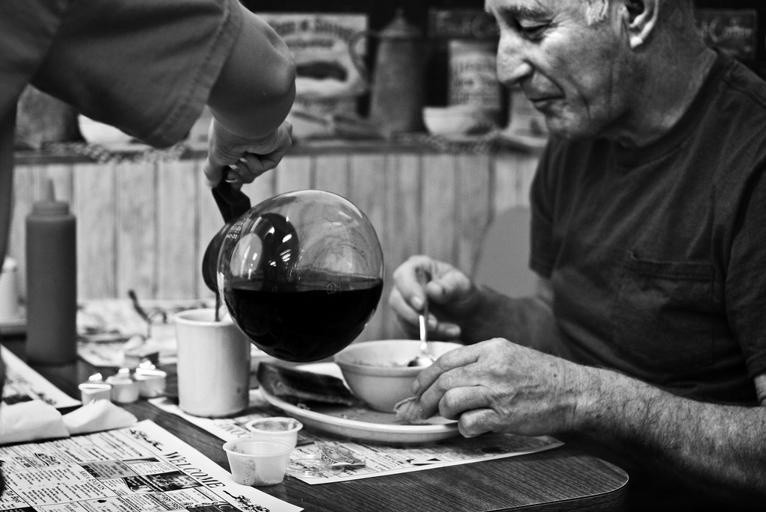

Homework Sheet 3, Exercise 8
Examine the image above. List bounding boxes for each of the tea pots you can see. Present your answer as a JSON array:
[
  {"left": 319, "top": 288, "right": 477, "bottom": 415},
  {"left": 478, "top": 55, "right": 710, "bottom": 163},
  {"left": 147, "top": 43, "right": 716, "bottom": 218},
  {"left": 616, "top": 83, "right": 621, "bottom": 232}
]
[{"left": 200, "top": 165, "right": 384, "bottom": 366}]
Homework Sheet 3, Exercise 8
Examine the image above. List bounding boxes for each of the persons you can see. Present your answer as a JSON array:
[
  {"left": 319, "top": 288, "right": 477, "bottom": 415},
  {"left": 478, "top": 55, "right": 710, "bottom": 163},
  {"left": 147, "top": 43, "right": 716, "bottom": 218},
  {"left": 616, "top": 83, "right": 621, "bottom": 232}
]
[
  {"left": 0, "top": 0, "right": 297, "bottom": 273},
  {"left": 388, "top": 0, "right": 766, "bottom": 512}
]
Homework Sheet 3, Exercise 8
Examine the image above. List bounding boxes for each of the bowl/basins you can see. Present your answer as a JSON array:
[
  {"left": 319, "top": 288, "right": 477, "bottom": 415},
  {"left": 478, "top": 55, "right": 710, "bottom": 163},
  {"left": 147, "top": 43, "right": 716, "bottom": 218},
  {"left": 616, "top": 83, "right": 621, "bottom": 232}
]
[{"left": 341, "top": 336, "right": 475, "bottom": 411}]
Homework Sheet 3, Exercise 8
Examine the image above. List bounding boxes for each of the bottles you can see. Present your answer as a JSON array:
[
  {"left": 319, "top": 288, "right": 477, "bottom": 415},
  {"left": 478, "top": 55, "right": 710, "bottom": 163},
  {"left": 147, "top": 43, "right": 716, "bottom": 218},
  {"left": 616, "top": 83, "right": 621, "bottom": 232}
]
[{"left": 26, "top": 174, "right": 78, "bottom": 365}]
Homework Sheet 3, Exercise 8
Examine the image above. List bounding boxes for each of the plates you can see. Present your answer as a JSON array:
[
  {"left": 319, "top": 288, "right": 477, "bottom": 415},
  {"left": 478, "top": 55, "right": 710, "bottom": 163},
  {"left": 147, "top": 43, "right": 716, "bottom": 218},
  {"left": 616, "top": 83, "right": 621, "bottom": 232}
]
[{"left": 263, "top": 376, "right": 484, "bottom": 441}]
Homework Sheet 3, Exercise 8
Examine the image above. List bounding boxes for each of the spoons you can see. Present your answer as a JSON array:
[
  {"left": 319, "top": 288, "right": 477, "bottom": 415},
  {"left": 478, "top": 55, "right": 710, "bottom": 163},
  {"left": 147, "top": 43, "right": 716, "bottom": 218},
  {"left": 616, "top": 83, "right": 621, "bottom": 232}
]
[{"left": 405, "top": 273, "right": 439, "bottom": 367}]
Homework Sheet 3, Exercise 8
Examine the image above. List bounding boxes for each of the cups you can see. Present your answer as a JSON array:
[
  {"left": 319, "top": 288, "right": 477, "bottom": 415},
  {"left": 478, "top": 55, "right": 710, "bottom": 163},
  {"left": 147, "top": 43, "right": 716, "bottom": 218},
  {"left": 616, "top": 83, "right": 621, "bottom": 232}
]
[
  {"left": 80, "top": 363, "right": 169, "bottom": 407},
  {"left": 175, "top": 306, "right": 249, "bottom": 418},
  {"left": 226, "top": 415, "right": 301, "bottom": 487},
  {"left": 425, "top": 103, "right": 483, "bottom": 139}
]
